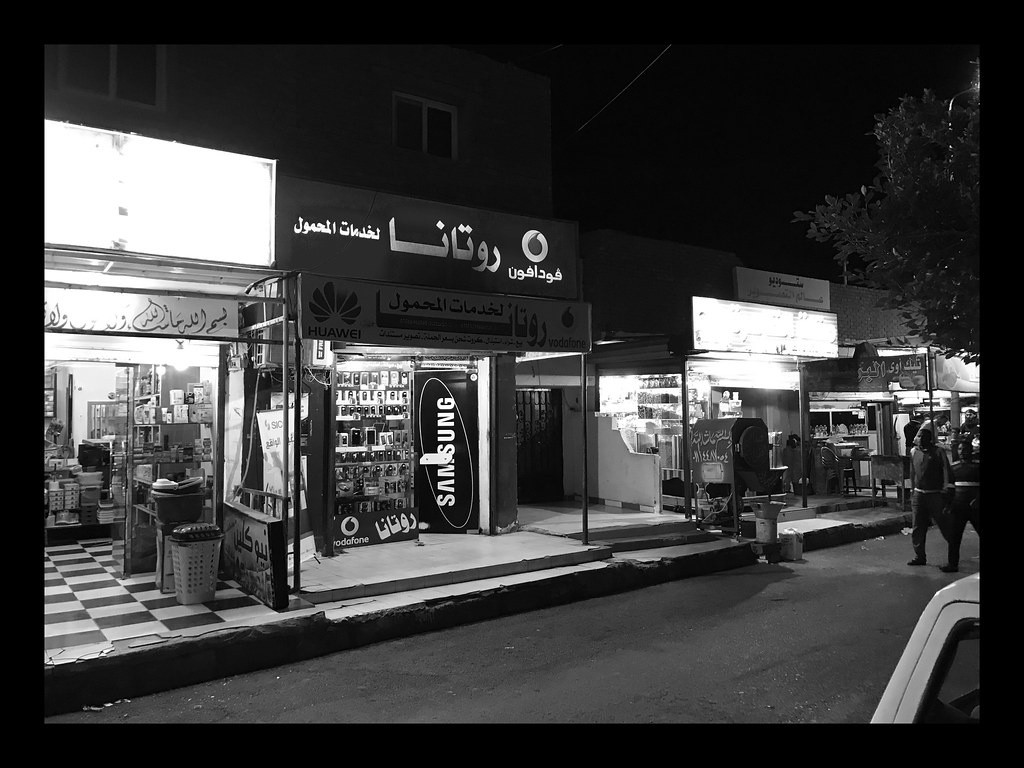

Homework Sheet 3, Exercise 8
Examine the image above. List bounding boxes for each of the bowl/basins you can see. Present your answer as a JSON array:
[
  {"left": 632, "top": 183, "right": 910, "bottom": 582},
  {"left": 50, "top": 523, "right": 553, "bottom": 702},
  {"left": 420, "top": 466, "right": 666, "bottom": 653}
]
[{"left": 78, "top": 471, "right": 103, "bottom": 485}]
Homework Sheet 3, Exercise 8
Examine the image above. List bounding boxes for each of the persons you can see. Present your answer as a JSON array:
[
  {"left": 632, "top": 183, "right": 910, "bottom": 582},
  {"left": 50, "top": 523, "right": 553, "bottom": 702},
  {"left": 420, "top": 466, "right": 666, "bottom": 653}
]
[{"left": 904, "top": 410, "right": 982, "bottom": 572}]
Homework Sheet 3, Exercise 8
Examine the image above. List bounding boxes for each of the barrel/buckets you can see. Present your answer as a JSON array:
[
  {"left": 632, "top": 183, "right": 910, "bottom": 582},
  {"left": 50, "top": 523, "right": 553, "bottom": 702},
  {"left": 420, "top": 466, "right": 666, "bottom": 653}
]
[{"left": 756, "top": 517, "right": 777, "bottom": 544}]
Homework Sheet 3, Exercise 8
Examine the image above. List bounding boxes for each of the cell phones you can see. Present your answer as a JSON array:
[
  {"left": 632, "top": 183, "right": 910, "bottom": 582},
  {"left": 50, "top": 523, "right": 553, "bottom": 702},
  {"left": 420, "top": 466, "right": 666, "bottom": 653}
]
[{"left": 335, "top": 371, "right": 412, "bottom": 517}]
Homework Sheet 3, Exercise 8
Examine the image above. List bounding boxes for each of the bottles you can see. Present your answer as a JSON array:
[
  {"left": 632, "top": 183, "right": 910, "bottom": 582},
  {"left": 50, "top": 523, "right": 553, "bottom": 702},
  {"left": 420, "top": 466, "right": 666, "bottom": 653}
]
[
  {"left": 815, "top": 424, "right": 827, "bottom": 437},
  {"left": 832, "top": 424, "right": 868, "bottom": 435}
]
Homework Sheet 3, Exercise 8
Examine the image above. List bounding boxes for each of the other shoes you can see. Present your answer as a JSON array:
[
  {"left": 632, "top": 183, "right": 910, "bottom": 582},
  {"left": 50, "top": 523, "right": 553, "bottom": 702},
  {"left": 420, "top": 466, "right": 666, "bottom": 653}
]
[
  {"left": 942, "top": 564, "right": 959, "bottom": 572},
  {"left": 907, "top": 556, "right": 927, "bottom": 565}
]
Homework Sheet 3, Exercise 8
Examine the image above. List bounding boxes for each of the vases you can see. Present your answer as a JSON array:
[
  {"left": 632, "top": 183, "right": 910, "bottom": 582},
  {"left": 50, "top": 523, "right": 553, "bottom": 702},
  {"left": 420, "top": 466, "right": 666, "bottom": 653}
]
[{"left": 748, "top": 499, "right": 786, "bottom": 544}]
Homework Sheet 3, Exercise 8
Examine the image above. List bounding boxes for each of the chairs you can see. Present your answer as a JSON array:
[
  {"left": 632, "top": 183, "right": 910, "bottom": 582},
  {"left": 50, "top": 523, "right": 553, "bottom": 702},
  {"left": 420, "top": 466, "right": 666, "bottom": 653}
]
[{"left": 820, "top": 446, "right": 858, "bottom": 497}]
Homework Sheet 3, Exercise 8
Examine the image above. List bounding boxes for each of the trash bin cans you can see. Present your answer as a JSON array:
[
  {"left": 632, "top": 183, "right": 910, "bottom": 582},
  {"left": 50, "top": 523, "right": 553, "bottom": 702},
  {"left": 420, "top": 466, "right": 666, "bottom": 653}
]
[
  {"left": 167, "top": 522, "right": 225, "bottom": 605},
  {"left": 778, "top": 533, "right": 804, "bottom": 561}
]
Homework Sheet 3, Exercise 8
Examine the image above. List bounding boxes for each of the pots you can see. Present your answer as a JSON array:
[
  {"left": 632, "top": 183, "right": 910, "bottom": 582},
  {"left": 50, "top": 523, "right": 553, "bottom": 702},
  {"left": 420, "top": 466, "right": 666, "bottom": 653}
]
[{"left": 749, "top": 500, "right": 786, "bottom": 519}]
